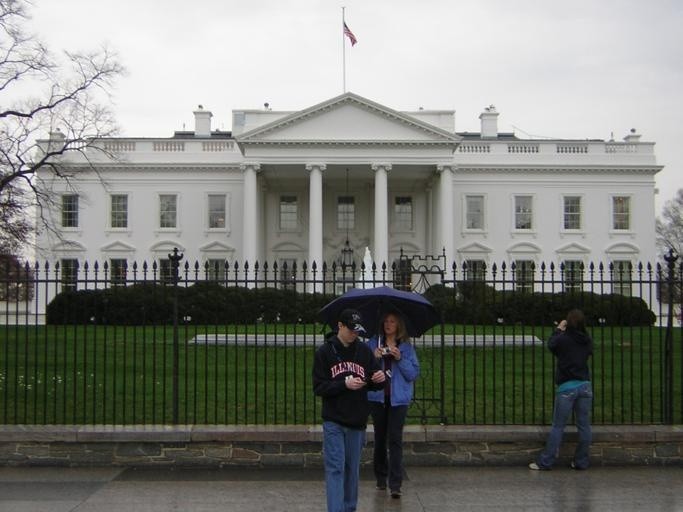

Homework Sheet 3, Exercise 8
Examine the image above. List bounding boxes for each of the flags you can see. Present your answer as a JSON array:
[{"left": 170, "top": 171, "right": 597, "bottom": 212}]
[{"left": 345, "top": 21, "right": 358, "bottom": 47}]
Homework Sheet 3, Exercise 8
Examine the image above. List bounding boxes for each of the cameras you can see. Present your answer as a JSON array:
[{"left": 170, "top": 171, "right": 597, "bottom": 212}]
[{"left": 381, "top": 347, "right": 391, "bottom": 355}]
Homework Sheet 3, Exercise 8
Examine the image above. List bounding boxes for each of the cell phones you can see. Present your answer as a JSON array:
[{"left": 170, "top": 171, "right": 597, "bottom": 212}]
[{"left": 362, "top": 376, "right": 373, "bottom": 382}]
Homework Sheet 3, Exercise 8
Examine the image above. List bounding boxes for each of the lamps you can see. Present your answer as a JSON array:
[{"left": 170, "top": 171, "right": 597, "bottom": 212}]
[{"left": 339, "top": 169, "right": 354, "bottom": 272}]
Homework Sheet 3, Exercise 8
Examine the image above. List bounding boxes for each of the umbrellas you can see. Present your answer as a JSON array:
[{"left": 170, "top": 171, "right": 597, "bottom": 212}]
[{"left": 314, "top": 285, "right": 445, "bottom": 348}]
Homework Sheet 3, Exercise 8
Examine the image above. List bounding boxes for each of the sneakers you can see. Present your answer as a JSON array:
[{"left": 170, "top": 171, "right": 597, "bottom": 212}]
[
  {"left": 375, "top": 475, "right": 387, "bottom": 490},
  {"left": 390, "top": 488, "right": 403, "bottom": 497},
  {"left": 528, "top": 463, "right": 540, "bottom": 469}
]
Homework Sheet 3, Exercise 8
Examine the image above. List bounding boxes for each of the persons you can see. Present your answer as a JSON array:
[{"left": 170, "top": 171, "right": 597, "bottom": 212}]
[
  {"left": 364, "top": 310, "right": 421, "bottom": 499},
  {"left": 528, "top": 308, "right": 594, "bottom": 470},
  {"left": 312, "top": 308, "right": 389, "bottom": 512}
]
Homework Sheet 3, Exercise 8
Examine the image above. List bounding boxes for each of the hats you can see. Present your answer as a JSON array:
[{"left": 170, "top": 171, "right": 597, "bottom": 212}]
[{"left": 338, "top": 308, "right": 367, "bottom": 334}]
